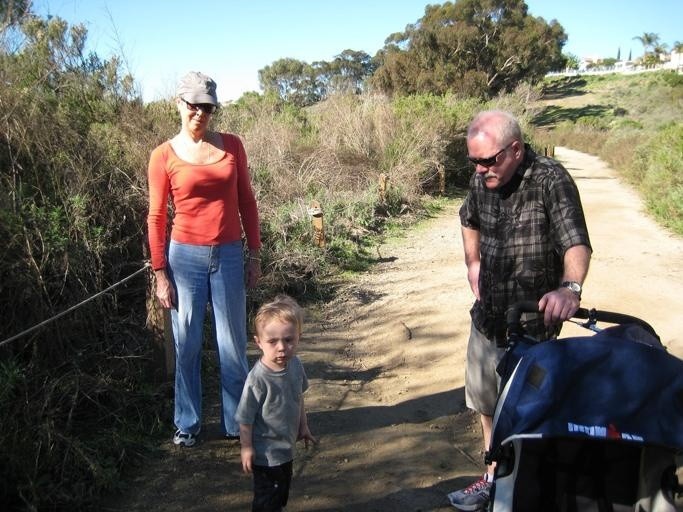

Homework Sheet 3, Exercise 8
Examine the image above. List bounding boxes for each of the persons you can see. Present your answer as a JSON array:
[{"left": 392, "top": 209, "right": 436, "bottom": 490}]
[
  {"left": 445, "top": 108, "right": 594, "bottom": 511},
  {"left": 233, "top": 292, "right": 316, "bottom": 512},
  {"left": 146, "top": 69, "right": 264, "bottom": 447}
]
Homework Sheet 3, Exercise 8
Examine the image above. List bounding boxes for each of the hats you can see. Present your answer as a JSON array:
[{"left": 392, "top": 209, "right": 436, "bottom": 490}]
[{"left": 176, "top": 71, "right": 218, "bottom": 107}]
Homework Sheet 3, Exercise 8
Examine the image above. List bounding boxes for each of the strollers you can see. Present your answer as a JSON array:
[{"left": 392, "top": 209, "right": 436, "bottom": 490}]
[{"left": 484, "top": 300, "right": 683, "bottom": 511}]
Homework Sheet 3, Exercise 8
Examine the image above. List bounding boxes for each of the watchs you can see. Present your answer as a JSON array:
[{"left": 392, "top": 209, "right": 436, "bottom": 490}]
[{"left": 559, "top": 280, "right": 582, "bottom": 301}]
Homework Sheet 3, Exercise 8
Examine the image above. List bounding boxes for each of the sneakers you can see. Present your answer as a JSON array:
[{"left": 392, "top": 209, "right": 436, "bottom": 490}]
[
  {"left": 445, "top": 472, "right": 495, "bottom": 511},
  {"left": 172, "top": 424, "right": 201, "bottom": 446}
]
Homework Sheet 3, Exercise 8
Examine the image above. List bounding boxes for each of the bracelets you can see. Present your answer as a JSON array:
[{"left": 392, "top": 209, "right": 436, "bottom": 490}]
[{"left": 245, "top": 254, "right": 262, "bottom": 263}]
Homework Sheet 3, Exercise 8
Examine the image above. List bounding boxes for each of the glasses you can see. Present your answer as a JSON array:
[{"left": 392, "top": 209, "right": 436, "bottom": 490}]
[
  {"left": 186, "top": 102, "right": 217, "bottom": 114},
  {"left": 466, "top": 141, "right": 513, "bottom": 167}
]
